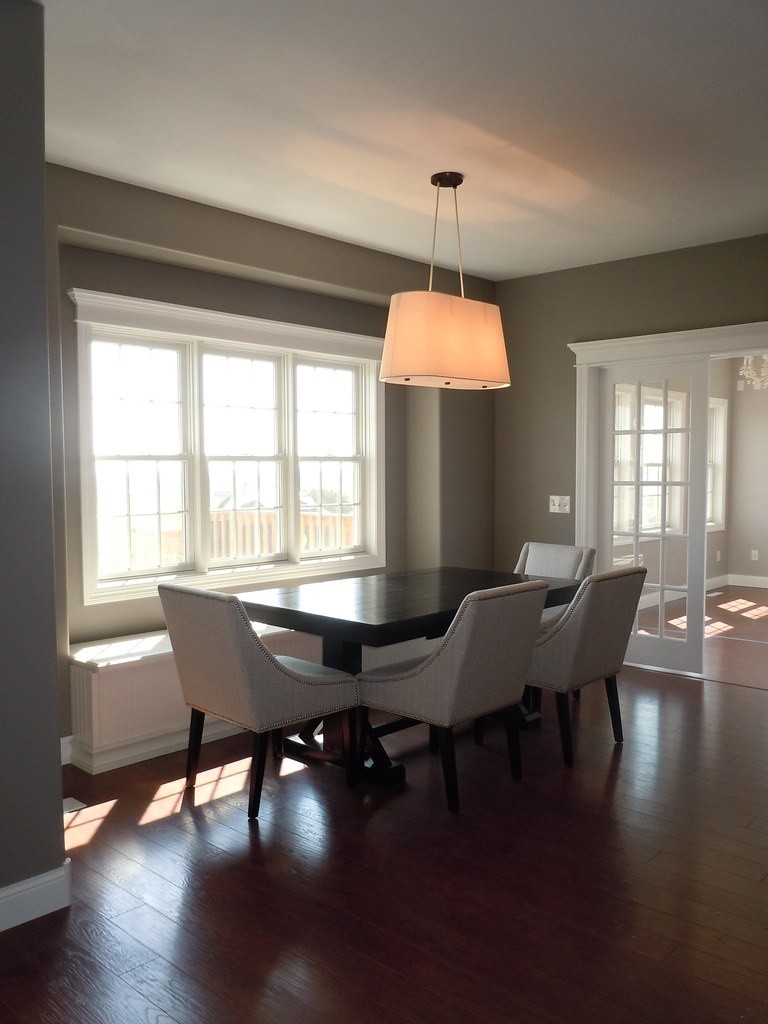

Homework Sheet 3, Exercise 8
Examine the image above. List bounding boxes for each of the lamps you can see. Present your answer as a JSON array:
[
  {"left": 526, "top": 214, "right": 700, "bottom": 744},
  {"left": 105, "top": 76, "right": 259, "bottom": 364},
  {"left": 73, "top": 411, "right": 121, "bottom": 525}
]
[
  {"left": 738, "top": 354, "right": 768, "bottom": 389},
  {"left": 378, "top": 172, "right": 513, "bottom": 390}
]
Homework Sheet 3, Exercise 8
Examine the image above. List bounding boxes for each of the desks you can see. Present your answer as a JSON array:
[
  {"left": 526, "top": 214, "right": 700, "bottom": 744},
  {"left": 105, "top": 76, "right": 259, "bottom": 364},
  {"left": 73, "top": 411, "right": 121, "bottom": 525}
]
[{"left": 229, "top": 568, "right": 582, "bottom": 782}]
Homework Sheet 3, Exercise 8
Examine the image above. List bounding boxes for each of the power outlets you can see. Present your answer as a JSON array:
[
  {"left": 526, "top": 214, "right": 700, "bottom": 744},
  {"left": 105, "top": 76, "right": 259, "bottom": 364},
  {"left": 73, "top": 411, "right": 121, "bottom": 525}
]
[{"left": 751, "top": 550, "right": 759, "bottom": 560}]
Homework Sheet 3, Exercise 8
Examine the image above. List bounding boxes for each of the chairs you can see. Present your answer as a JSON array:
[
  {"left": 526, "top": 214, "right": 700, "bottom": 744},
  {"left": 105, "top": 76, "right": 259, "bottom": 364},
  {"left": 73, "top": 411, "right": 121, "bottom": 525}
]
[
  {"left": 517, "top": 567, "right": 648, "bottom": 766},
  {"left": 513, "top": 542, "right": 597, "bottom": 699},
  {"left": 157, "top": 584, "right": 362, "bottom": 818},
  {"left": 352, "top": 580, "right": 549, "bottom": 812}
]
